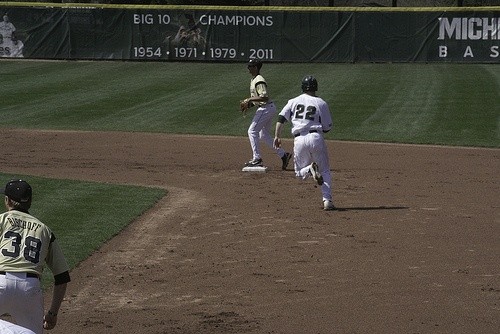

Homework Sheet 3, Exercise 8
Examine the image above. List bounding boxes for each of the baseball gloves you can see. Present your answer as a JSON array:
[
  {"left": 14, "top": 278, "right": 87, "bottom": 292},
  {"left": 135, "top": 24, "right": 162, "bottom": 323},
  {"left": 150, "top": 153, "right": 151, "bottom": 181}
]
[{"left": 239, "top": 99, "right": 254, "bottom": 112}]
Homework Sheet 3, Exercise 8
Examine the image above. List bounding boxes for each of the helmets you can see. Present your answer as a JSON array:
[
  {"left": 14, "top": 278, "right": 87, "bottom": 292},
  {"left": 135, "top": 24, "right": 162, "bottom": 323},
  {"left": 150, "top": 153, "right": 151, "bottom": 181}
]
[{"left": 301, "top": 75, "right": 319, "bottom": 93}]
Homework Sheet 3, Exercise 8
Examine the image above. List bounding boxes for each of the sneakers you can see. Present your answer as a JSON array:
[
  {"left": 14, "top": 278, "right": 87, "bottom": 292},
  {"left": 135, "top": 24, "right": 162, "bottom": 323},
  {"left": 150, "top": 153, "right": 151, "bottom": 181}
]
[
  {"left": 323, "top": 200, "right": 337, "bottom": 211},
  {"left": 245, "top": 159, "right": 264, "bottom": 168},
  {"left": 280, "top": 153, "right": 293, "bottom": 170},
  {"left": 308, "top": 161, "right": 324, "bottom": 186}
]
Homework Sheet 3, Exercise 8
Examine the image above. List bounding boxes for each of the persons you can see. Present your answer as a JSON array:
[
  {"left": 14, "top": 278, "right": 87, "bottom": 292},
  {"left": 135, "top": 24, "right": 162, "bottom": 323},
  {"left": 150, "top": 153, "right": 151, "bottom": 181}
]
[
  {"left": 272, "top": 75, "right": 336, "bottom": 211},
  {"left": 240, "top": 58, "right": 293, "bottom": 171},
  {"left": 0, "top": 179, "right": 71, "bottom": 333},
  {"left": 0, "top": 11, "right": 17, "bottom": 48},
  {"left": 0, "top": 31, "right": 13, "bottom": 58},
  {"left": 8, "top": 33, "right": 26, "bottom": 58}
]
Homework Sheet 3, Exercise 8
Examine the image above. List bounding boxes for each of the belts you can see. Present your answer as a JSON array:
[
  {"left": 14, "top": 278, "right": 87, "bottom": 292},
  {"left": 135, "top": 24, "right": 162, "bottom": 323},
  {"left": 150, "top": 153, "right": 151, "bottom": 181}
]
[
  {"left": 0, "top": 271, "right": 39, "bottom": 281},
  {"left": 293, "top": 129, "right": 323, "bottom": 138}
]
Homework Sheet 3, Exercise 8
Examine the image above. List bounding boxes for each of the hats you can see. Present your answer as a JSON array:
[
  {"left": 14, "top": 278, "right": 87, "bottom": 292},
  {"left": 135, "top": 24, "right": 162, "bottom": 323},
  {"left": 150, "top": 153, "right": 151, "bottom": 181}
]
[
  {"left": 247, "top": 58, "right": 263, "bottom": 69},
  {"left": 0, "top": 179, "right": 34, "bottom": 201}
]
